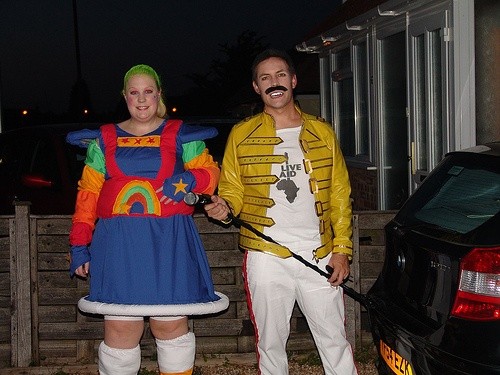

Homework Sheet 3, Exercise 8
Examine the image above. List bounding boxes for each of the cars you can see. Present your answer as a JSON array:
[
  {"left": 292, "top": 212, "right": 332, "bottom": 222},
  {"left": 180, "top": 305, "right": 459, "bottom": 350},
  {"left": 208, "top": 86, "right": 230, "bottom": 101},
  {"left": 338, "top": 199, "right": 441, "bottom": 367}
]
[{"left": 365, "top": 140, "right": 500, "bottom": 375}]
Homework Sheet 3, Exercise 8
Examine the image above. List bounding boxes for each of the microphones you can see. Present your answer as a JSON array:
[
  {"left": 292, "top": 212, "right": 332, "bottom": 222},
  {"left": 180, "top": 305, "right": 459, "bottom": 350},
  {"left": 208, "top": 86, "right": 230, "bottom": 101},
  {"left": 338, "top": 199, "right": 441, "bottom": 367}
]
[{"left": 183, "top": 192, "right": 213, "bottom": 205}]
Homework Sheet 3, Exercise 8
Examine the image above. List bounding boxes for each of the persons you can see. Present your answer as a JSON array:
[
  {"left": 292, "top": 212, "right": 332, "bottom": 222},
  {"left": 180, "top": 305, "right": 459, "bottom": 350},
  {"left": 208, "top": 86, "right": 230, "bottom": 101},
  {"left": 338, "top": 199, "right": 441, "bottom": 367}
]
[
  {"left": 68, "top": 65, "right": 230, "bottom": 375},
  {"left": 202, "top": 47, "right": 360, "bottom": 374}
]
